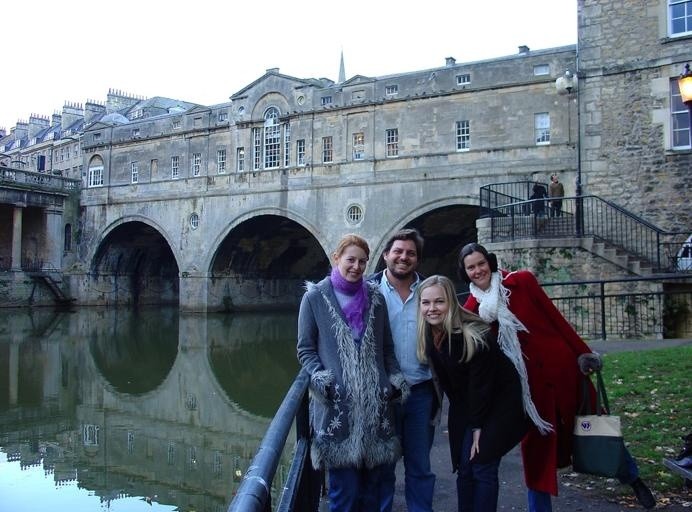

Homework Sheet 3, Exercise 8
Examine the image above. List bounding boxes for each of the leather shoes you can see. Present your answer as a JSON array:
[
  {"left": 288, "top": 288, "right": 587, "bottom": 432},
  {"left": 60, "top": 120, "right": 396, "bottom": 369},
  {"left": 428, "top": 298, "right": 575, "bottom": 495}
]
[{"left": 629, "top": 476, "right": 656, "bottom": 508}]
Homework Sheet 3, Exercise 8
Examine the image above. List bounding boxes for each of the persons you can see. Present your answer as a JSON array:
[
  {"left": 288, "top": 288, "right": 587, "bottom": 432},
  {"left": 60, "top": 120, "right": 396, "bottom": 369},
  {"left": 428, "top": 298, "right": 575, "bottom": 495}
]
[
  {"left": 532, "top": 174, "right": 564, "bottom": 217},
  {"left": 459, "top": 243, "right": 657, "bottom": 512},
  {"left": 296, "top": 235, "right": 410, "bottom": 512},
  {"left": 362, "top": 230, "right": 444, "bottom": 512},
  {"left": 416, "top": 275, "right": 530, "bottom": 512}
]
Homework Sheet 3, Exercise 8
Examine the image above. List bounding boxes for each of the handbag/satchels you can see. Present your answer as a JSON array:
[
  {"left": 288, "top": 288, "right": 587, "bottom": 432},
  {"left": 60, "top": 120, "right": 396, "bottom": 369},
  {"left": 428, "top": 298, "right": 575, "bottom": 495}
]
[{"left": 573, "top": 366, "right": 623, "bottom": 477}]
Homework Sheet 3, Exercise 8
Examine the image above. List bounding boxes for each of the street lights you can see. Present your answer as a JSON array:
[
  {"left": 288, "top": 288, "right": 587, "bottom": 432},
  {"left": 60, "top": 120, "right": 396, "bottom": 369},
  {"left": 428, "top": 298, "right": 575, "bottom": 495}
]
[
  {"left": 565, "top": 51, "right": 584, "bottom": 238},
  {"left": 48, "top": 142, "right": 55, "bottom": 187}
]
